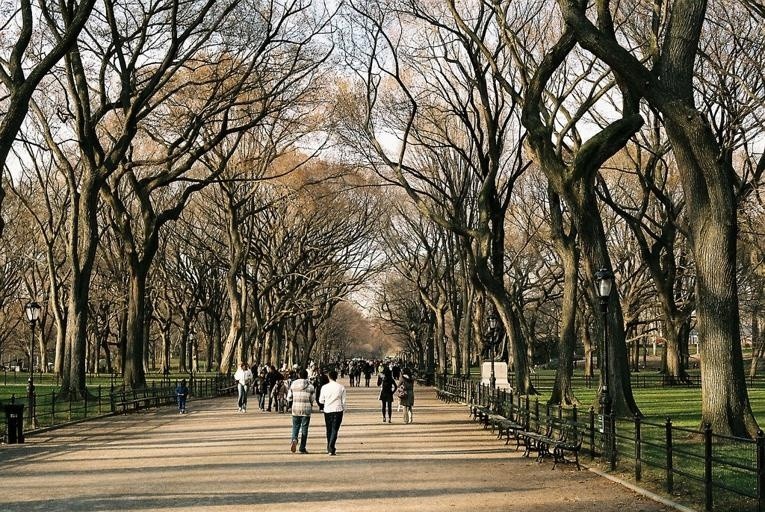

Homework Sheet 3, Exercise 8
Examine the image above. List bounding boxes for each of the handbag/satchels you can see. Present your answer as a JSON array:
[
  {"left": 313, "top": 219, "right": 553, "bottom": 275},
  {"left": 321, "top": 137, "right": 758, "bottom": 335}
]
[{"left": 397, "top": 385, "right": 407, "bottom": 398}]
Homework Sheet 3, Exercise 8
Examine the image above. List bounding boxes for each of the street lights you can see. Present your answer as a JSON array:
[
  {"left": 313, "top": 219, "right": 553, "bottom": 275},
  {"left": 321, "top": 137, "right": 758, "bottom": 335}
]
[
  {"left": 594, "top": 265, "right": 618, "bottom": 461},
  {"left": 23, "top": 298, "right": 42, "bottom": 428},
  {"left": 257, "top": 341, "right": 341, "bottom": 367},
  {"left": 400, "top": 334, "right": 450, "bottom": 386},
  {"left": 188, "top": 329, "right": 196, "bottom": 377},
  {"left": 487, "top": 313, "right": 497, "bottom": 397}
]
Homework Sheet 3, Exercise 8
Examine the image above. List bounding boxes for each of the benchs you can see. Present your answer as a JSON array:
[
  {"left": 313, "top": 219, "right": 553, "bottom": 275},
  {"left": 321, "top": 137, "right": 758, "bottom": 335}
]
[
  {"left": 435, "top": 382, "right": 461, "bottom": 404},
  {"left": 473, "top": 395, "right": 587, "bottom": 472},
  {"left": 212, "top": 379, "right": 238, "bottom": 397},
  {"left": 415, "top": 374, "right": 428, "bottom": 386},
  {"left": 109, "top": 387, "right": 180, "bottom": 415}
]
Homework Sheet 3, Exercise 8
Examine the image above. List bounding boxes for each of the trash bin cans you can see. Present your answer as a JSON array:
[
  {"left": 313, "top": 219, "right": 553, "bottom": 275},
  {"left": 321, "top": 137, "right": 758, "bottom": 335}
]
[{"left": 0, "top": 404, "right": 24, "bottom": 443}]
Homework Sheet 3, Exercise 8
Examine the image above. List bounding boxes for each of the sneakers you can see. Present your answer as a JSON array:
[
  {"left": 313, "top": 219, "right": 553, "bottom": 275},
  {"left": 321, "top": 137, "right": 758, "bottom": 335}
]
[
  {"left": 299, "top": 451, "right": 308, "bottom": 453},
  {"left": 291, "top": 440, "right": 297, "bottom": 452},
  {"left": 239, "top": 407, "right": 246, "bottom": 412},
  {"left": 329, "top": 452, "right": 336, "bottom": 454},
  {"left": 258, "top": 408, "right": 278, "bottom": 412}
]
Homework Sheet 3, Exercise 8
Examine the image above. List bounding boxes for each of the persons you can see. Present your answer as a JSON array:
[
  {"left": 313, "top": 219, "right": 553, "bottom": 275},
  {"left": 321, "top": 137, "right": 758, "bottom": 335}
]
[
  {"left": 286, "top": 368, "right": 315, "bottom": 453},
  {"left": 334, "top": 356, "right": 403, "bottom": 386},
  {"left": 233, "top": 357, "right": 329, "bottom": 413},
  {"left": 175, "top": 378, "right": 189, "bottom": 415},
  {"left": 318, "top": 371, "right": 347, "bottom": 455},
  {"left": 375, "top": 366, "right": 397, "bottom": 423},
  {"left": 396, "top": 368, "right": 415, "bottom": 424}
]
